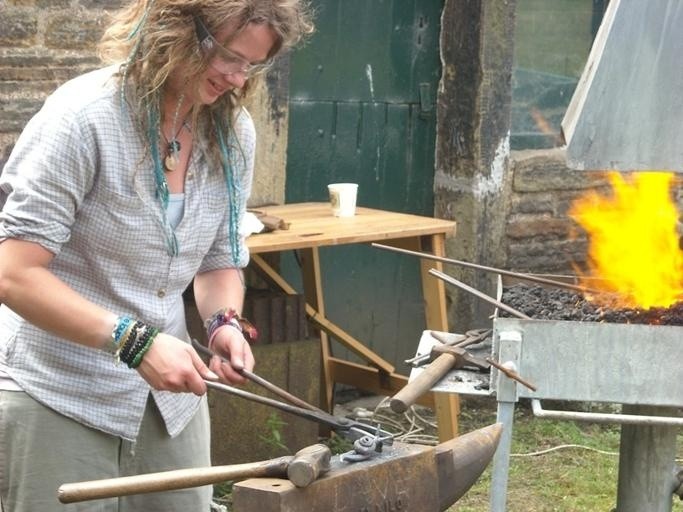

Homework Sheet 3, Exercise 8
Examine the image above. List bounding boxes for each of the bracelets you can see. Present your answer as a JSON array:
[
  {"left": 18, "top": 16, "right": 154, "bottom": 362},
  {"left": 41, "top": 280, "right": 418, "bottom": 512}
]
[
  {"left": 205, "top": 310, "right": 257, "bottom": 347},
  {"left": 111, "top": 317, "right": 158, "bottom": 370}
]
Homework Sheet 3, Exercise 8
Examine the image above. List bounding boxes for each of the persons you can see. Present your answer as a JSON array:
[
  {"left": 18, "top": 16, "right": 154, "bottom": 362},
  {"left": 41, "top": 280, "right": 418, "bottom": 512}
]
[{"left": 0, "top": 1, "right": 313, "bottom": 512}]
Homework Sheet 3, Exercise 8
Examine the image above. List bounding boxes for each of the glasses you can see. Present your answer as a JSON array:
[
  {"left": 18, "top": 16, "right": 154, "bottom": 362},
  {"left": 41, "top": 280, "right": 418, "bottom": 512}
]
[{"left": 192, "top": 12, "right": 275, "bottom": 78}]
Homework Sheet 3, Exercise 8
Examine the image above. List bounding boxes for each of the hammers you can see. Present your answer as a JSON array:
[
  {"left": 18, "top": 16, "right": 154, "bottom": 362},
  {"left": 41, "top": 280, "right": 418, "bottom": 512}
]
[
  {"left": 391, "top": 345, "right": 492, "bottom": 414},
  {"left": 58, "top": 443, "right": 331, "bottom": 505}
]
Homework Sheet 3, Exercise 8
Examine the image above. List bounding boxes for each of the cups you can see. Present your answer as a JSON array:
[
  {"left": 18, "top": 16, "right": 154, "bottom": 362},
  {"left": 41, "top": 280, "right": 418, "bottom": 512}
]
[{"left": 326, "top": 182, "right": 360, "bottom": 217}]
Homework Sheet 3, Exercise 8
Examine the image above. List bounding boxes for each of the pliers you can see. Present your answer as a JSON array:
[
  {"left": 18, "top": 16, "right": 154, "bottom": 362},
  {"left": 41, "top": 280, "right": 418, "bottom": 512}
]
[
  {"left": 404, "top": 328, "right": 493, "bottom": 368},
  {"left": 192, "top": 338, "right": 394, "bottom": 453}
]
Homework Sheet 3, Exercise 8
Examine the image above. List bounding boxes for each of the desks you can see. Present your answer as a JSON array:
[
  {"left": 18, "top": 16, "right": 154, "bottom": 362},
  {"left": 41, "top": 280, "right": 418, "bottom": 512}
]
[{"left": 239, "top": 202, "right": 462, "bottom": 447}]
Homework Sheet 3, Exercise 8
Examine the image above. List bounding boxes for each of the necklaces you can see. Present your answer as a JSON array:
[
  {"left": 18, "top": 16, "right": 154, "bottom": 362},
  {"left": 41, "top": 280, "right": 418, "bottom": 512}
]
[{"left": 152, "top": 112, "right": 194, "bottom": 171}]
